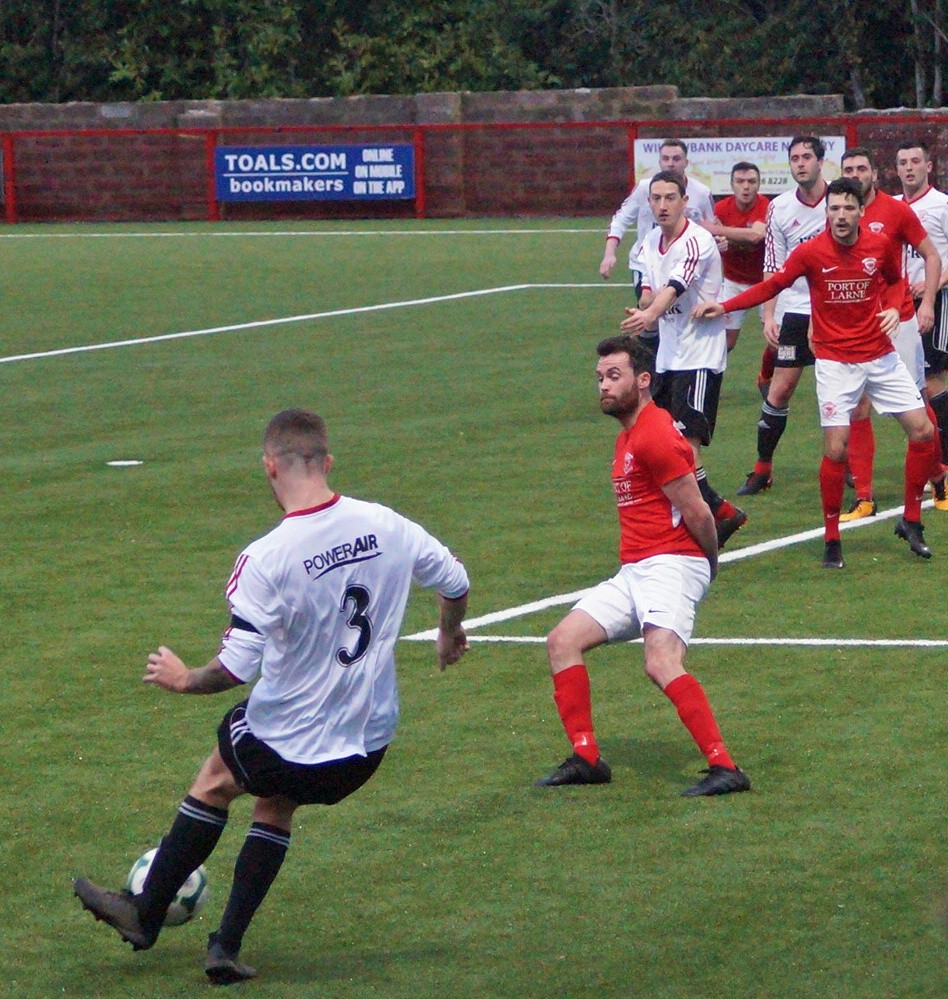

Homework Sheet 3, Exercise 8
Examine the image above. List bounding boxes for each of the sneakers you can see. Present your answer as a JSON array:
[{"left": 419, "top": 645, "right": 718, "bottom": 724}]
[
  {"left": 680, "top": 764, "right": 750, "bottom": 797},
  {"left": 206, "top": 932, "right": 257, "bottom": 984},
  {"left": 73, "top": 877, "right": 166, "bottom": 951},
  {"left": 535, "top": 752, "right": 612, "bottom": 786},
  {"left": 737, "top": 472, "right": 774, "bottom": 495},
  {"left": 823, "top": 538, "right": 845, "bottom": 569},
  {"left": 894, "top": 517, "right": 932, "bottom": 558},
  {"left": 929, "top": 471, "right": 948, "bottom": 510},
  {"left": 710, "top": 496, "right": 748, "bottom": 554},
  {"left": 839, "top": 499, "right": 877, "bottom": 522}
]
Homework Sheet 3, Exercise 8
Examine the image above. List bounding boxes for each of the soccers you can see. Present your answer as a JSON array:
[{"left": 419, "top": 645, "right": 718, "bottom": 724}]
[{"left": 126, "top": 848, "right": 211, "bottom": 927}]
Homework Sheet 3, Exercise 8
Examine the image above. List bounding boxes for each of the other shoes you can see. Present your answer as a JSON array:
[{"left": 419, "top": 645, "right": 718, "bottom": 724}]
[
  {"left": 756, "top": 372, "right": 773, "bottom": 400},
  {"left": 846, "top": 467, "right": 855, "bottom": 488}
]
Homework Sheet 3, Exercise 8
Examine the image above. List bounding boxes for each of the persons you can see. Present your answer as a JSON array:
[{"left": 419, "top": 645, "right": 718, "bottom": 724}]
[
  {"left": 600, "top": 136, "right": 948, "bottom": 568},
  {"left": 71, "top": 408, "right": 470, "bottom": 986},
  {"left": 537, "top": 335, "right": 748, "bottom": 798}
]
